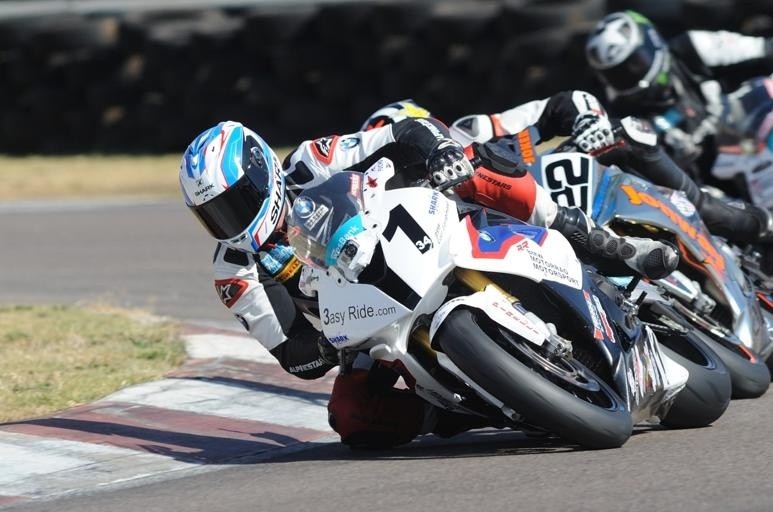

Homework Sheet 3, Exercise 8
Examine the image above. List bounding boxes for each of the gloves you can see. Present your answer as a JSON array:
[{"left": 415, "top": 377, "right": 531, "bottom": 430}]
[
  {"left": 427, "top": 138, "right": 476, "bottom": 187},
  {"left": 315, "top": 335, "right": 360, "bottom": 365},
  {"left": 570, "top": 113, "right": 617, "bottom": 156}
]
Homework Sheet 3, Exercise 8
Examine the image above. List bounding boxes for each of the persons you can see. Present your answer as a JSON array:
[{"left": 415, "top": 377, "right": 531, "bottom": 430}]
[
  {"left": 582, "top": 10, "right": 772, "bottom": 153},
  {"left": 176, "top": 114, "right": 681, "bottom": 449},
  {"left": 357, "top": 89, "right": 762, "bottom": 246}
]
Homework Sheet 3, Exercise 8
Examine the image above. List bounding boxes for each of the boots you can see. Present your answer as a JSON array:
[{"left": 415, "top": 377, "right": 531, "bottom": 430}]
[
  {"left": 548, "top": 204, "right": 680, "bottom": 279},
  {"left": 678, "top": 170, "right": 766, "bottom": 241}
]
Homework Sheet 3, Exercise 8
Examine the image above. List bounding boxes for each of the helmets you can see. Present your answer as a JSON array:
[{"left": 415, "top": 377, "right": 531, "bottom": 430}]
[
  {"left": 584, "top": 10, "right": 670, "bottom": 95},
  {"left": 178, "top": 118, "right": 287, "bottom": 254}
]
